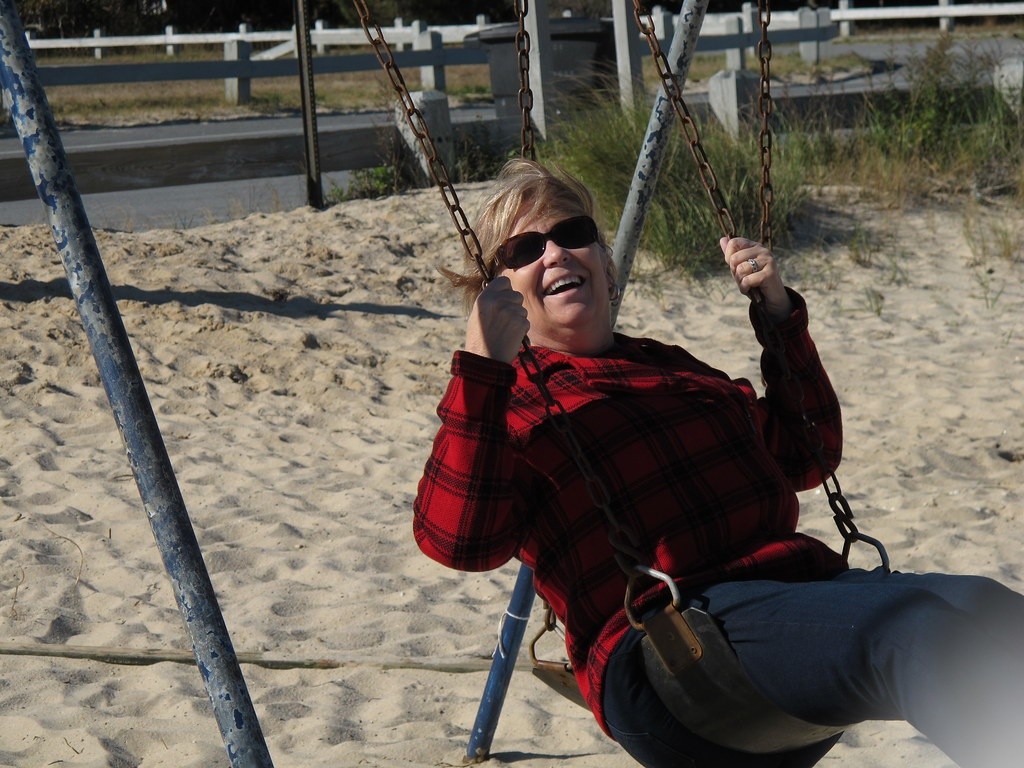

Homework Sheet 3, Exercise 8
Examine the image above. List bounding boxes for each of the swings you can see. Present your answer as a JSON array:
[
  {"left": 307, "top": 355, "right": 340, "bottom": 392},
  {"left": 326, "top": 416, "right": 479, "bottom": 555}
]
[
  {"left": 346, "top": 0, "right": 892, "bottom": 756},
  {"left": 513, "top": 0, "right": 772, "bottom": 715}
]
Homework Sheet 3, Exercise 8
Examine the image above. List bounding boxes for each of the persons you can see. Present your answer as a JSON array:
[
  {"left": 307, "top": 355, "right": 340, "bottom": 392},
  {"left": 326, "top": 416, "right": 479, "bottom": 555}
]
[{"left": 411, "top": 158, "right": 1024, "bottom": 768}]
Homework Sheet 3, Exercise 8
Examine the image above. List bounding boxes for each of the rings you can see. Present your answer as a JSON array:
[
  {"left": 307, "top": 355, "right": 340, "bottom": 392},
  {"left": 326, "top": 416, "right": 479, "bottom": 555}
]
[{"left": 748, "top": 258, "right": 759, "bottom": 273}]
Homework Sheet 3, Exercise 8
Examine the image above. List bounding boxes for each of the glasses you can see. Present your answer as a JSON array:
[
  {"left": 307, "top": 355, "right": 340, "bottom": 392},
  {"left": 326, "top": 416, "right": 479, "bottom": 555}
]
[{"left": 489, "top": 214, "right": 606, "bottom": 274}]
